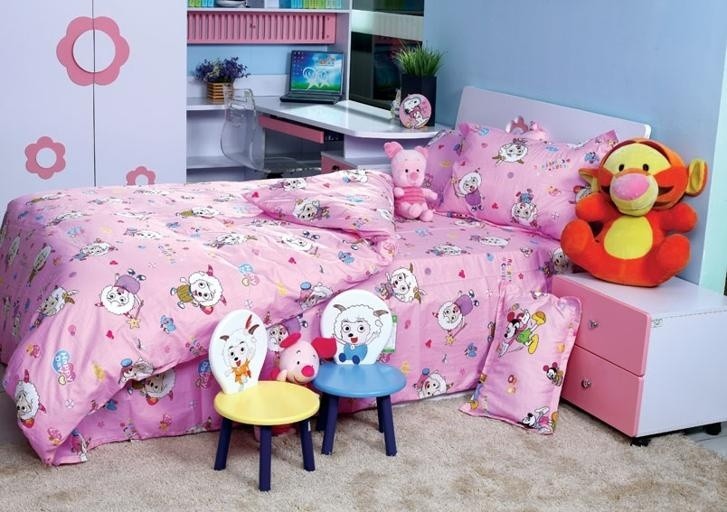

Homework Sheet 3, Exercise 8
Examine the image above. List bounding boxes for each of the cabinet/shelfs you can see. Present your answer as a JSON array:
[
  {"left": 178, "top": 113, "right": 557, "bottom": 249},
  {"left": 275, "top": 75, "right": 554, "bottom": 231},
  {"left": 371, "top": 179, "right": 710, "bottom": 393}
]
[{"left": 0, "top": 1, "right": 187, "bottom": 234}]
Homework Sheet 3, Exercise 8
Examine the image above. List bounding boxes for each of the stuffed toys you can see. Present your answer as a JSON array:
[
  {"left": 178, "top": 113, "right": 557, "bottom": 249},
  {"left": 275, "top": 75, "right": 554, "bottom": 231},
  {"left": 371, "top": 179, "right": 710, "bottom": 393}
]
[
  {"left": 383, "top": 140, "right": 438, "bottom": 222},
  {"left": 559, "top": 137, "right": 708, "bottom": 287},
  {"left": 254, "top": 332, "right": 340, "bottom": 443}
]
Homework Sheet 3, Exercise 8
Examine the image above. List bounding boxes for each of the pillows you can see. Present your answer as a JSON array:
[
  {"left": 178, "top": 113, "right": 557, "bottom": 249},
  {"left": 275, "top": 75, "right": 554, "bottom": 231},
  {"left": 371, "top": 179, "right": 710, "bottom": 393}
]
[
  {"left": 432, "top": 118, "right": 620, "bottom": 242},
  {"left": 419, "top": 125, "right": 463, "bottom": 210},
  {"left": 456, "top": 279, "right": 585, "bottom": 436}
]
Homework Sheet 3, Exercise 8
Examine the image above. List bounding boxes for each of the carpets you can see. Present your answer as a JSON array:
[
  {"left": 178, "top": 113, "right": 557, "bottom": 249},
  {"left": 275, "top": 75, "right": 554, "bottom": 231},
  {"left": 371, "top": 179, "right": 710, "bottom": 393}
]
[{"left": 0, "top": 392, "right": 726, "bottom": 512}]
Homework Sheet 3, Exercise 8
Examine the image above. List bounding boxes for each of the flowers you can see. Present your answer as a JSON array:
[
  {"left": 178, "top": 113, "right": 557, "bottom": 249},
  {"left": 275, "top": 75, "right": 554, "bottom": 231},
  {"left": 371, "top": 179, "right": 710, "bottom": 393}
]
[{"left": 194, "top": 55, "right": 254, "bottom": 83}]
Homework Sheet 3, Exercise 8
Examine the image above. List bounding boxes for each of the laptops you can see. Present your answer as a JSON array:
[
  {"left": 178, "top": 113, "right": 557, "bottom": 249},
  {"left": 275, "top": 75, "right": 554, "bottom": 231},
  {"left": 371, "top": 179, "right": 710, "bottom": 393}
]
[{"left": 280, "top": 50, "right": 344, "bottom": 105}]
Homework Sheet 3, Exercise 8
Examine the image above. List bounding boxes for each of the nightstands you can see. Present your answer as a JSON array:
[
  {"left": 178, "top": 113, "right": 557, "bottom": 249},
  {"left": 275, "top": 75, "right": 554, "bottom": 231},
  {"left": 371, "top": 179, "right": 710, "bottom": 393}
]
[{"left": 550, "top": 271, "right": 726, "bottom": 448}]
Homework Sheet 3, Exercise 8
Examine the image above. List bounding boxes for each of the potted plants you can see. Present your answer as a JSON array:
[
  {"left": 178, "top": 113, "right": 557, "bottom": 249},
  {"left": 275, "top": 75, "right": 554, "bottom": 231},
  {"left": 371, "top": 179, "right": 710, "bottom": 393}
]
[{"left": 390, "top": 39, "right": 451, "bottom": 128}]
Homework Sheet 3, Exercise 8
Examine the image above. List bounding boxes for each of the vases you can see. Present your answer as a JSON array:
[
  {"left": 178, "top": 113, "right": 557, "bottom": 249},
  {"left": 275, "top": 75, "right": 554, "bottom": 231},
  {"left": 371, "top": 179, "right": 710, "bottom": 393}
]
[{"left": 207, "top": 79, "right": 235, "bottom": 102}]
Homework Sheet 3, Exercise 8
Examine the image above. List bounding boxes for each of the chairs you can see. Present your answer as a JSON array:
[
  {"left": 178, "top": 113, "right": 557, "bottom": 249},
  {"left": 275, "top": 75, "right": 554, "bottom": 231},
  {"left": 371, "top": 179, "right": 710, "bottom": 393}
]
[
  {"left": 309, "top": 286, "right": 409, "bottom": 457},
  {"left": 219, "top": 84, "right": 322, "bottom": 179},
  {"left": 207, "top": 307, "right": 321, "bottom": 492}
]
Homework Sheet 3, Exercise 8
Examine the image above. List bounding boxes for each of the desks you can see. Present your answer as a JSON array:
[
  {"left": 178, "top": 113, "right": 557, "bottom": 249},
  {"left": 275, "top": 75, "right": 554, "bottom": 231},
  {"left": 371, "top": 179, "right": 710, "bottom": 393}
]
[{"left": 184, "top": 94, "right": 453, "bottom": 174}]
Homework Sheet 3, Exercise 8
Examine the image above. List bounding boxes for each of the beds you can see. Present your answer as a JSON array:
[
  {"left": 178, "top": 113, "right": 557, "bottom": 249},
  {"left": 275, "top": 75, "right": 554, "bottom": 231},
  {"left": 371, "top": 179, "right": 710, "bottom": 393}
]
[{"left": 0, "top": 85, "right": 653, "bottom": 471}]
